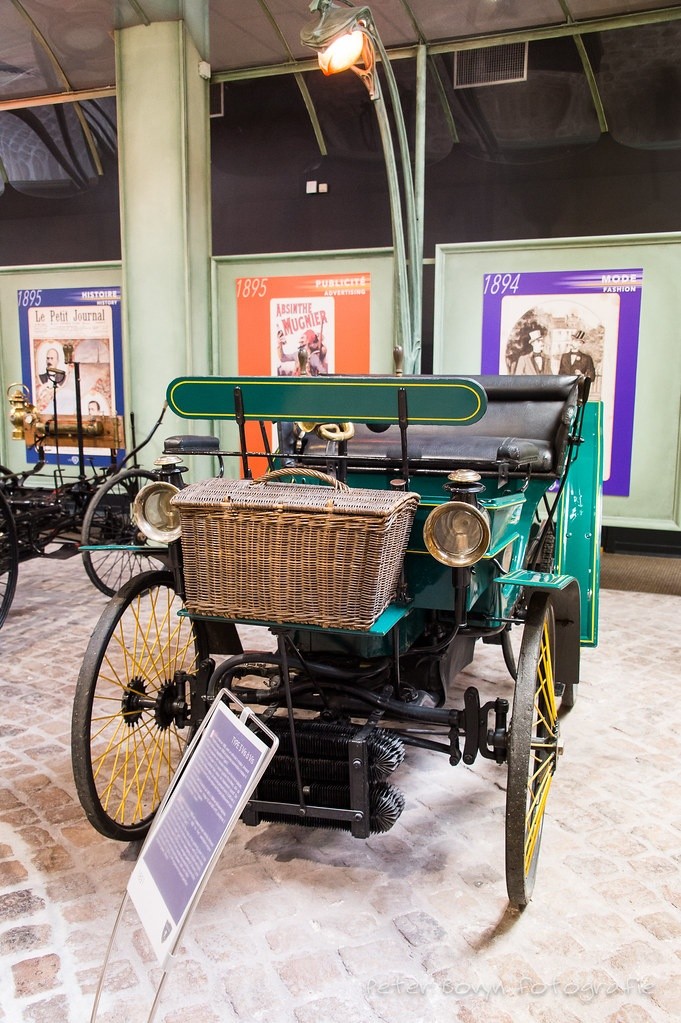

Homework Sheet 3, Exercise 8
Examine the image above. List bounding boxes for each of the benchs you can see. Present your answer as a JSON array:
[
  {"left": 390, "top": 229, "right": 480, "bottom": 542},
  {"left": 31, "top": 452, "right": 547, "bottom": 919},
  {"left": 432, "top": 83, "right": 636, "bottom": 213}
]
[{"left": 283, "top": 374, "right": 581, "bottom": 478}]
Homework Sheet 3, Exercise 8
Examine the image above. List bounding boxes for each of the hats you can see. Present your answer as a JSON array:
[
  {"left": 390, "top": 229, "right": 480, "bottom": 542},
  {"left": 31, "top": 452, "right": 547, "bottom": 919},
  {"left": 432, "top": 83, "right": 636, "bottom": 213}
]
[
  {"left": 571, "top": 330, "right": 587, "bottom": 344},
  {"left": 528, "top": 329, "right": 544, "bottom": 344}
]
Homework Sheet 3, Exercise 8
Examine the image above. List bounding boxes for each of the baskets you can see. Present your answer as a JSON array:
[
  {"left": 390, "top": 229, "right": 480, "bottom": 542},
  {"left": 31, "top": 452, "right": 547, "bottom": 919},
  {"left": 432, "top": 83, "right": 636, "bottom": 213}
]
[{"left": 168, "top": 467, "right": 422, "bottom": 632}]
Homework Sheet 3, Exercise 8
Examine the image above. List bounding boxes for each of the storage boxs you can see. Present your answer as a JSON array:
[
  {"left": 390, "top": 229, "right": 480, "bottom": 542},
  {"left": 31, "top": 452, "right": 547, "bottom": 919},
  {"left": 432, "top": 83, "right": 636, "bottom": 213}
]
[{"left": 170, "top": 468, "right": 421, "bottom": 630}]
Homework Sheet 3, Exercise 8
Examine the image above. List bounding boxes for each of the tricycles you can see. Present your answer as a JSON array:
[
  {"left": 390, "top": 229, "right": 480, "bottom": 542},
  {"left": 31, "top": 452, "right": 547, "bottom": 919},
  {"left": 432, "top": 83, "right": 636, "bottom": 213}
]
[
  {"left": 1, "top": 343, "right": 185, "bottom": 634},
  {"left": 71, "top": 345, "right": 603, "bottom": 908}
]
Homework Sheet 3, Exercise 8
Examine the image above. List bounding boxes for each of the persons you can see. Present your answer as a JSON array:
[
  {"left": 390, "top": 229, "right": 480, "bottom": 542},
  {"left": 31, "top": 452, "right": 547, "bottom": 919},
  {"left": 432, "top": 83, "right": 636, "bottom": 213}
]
[
  {"left": 559, "top": 330, "right": 596, "bottom": 382},
  {"left": 39, "top": 349, "right": 65, "bottom": 389},
  {"left": 277, "top": 329, "right": 328, "bottom": 377},
  {"left": 514, "top": 330, "right": 554, "bottom": 376},
  {"left": 87, "top": 401, "right": 100, "bottom": 416}
]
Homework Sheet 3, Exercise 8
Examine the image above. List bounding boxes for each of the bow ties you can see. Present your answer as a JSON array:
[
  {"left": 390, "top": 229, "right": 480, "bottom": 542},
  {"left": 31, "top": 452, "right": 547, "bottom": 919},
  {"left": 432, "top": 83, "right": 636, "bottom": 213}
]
[
  {"left": 533, "top": 352, "right": 542, "bottom": 358},
  {"left": 569, "top": 352, "right": 582, "bottom": 356},
  {"left": 48, "top": 374, "right": 56, "bottom": 378}
]
